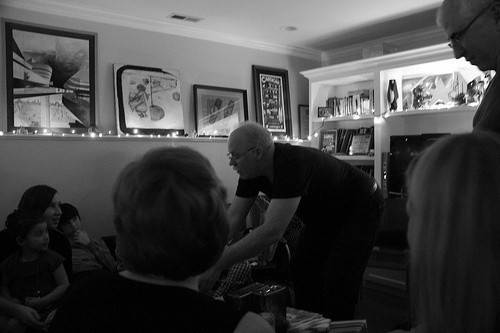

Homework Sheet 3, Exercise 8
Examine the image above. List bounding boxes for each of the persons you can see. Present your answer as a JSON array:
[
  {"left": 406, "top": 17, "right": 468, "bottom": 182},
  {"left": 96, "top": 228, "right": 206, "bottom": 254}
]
[
  {"left": 0, "top": 209, "right": 70, "bottom": 333},
  {"left": 0, "top": 185, "right": 71, "bottom": 332},
  {"left": 58, "top": 204, "right": 114, "bottom": 285},
  {"left": 390, "top": 129, "right": 500, "bottom": 332},
  {"left": 63, "top": 147, "right": 275, "bottom": 333},
  {"left": 435, "top": 0, "right": 500, "bottom": 133},
  {"left": 200, "top": 120, "right": 390, "bottom": 320}
]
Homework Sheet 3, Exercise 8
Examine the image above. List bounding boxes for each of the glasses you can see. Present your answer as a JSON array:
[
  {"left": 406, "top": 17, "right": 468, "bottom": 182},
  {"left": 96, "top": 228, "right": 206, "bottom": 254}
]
[
  {"left": 227, "top": 147, "right": 260, "bottom": 160},
  {"left": 449, "top": 7, "right": 487, "bottom": 51}
]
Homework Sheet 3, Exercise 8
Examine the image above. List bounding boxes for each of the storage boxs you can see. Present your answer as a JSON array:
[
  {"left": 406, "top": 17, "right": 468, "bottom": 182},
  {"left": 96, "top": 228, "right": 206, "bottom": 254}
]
[{"left": 225, "top": 283, "right": 268, "bottom": 310}]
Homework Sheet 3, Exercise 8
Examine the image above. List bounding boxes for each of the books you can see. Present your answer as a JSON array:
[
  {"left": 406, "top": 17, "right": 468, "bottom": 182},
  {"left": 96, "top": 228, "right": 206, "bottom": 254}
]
[{"left": 327, "top": 90, "right": 375, "bottom": 155}]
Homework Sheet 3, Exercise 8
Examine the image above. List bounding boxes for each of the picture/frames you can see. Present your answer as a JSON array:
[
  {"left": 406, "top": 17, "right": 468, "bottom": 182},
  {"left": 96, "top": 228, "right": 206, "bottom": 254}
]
[
  {"left": 4, "top": 21, "right": 96, "bottom": 133},
  {"left": 320, "top": 130, "right": 338, "bottom": 154},
  {"left": 113, "top": 64, "right": 185, "bottom": 135},
  {"left": 193, "top": 84, "right": 249, "bottom": 137},
  {"left": 251, "top": 64, "right": 293, "bottom": 139}
]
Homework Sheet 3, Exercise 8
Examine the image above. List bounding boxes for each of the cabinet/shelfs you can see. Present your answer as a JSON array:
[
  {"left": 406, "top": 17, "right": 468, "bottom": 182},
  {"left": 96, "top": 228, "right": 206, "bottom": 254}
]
[{"left": 298, "top": 43, "right": 496, "bottom": 200}]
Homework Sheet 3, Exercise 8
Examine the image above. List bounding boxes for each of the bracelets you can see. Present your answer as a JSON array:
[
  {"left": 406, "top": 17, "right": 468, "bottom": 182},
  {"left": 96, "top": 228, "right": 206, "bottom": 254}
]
[{"left": 38, "top": 296, "right": 41, "bottom": 308}]
